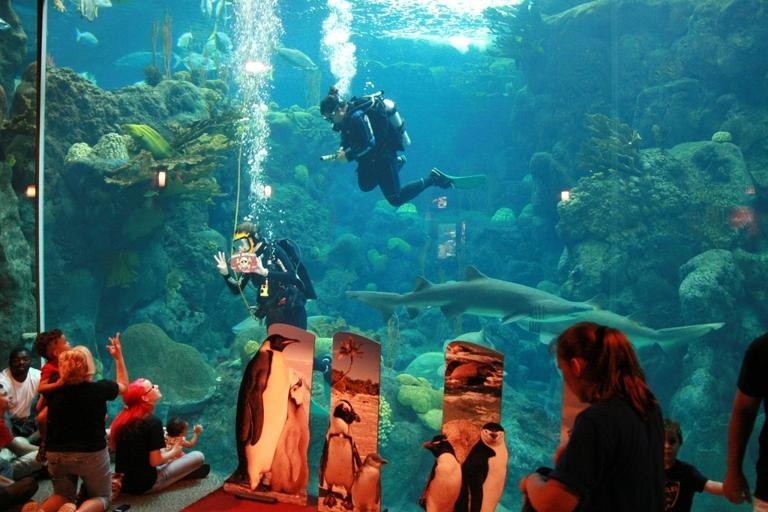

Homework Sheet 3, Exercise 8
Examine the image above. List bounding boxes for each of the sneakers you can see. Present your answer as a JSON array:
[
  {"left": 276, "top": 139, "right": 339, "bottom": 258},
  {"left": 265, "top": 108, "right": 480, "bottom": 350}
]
[
  {"left": 187, "top": 464, "right": 211, "bottom": 479},
  {"left": 36, "top": 447, "right": 45, "bottom": 463}
]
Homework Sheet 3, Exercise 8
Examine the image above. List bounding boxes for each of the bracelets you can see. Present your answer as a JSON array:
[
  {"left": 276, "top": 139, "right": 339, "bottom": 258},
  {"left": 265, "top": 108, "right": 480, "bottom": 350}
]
[{"left": 525, "top": 471, "right": 542, "bottom": 479}]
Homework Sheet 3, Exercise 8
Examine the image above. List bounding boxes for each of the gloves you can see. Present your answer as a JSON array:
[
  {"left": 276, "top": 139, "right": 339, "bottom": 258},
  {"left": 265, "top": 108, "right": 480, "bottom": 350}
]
[
  {"left": 214, "top": 251, "right": 229, "bottom": 276},
  {"left": 253, "top": 259, "right": 269, "bottom": 277}
]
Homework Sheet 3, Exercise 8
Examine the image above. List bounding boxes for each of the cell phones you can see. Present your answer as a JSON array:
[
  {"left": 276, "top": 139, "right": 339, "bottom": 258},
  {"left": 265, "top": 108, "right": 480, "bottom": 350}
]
[{"left": 114, "top": 504, "right": 130, "bottom": 512}]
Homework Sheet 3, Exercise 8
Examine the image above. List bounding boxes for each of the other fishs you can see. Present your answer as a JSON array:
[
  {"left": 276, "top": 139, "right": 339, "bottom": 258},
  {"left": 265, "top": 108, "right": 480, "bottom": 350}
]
[
  {"left": 113, "top": 51, "right": 167, "bottom": 71},
  {"left": 270, "top": 37, "right": 318, "bottom": 72},
  {"left": 171, "top": 0, "right": 232, "bottom": 73},
  {"left": 55, "top": 0, "right": 112, "bottom": 45},
  {"left": 122, "top": 124, "right": 183, "bottom": 161},
  {"left": 539, "top": 1, "right": 609, "bottom": 28}
]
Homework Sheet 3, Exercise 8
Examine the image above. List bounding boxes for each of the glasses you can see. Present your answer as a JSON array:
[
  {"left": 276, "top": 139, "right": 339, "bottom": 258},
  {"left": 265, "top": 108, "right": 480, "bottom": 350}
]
[
  {"left": 145, "top": 383, "right": 155, "bottom": 396},
  {"left": 232, "top": 238, "right": 254, "bottom": 254},
  {"left": 323, "top": 105, "right": 340, "bottom": 122}
]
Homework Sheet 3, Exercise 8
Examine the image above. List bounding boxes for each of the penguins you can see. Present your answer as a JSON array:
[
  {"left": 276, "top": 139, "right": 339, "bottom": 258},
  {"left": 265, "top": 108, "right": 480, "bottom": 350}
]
[
  {"left": 236, "top": 334, "right": 301, "bottom": 491},
  {"left": 419, "top": 434, "right": 463, "bottom": 512},
  {"left": 462, "top": 422, "right": 508, "bottom": 512},
  {"left": 270, "top": 372, "right": 310, "bottom": 497},
  {"left": 348, "top": 453, "right": 388, "bottom": 512},
  {"left": 318, "top": 400, "right": 362, "bottom": 511}
]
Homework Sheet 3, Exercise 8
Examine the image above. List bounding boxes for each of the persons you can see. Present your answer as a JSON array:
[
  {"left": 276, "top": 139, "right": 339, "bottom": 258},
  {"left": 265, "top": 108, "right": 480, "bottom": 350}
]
[
  {"left": 317, "top": 83, "right": 453, "bottom": 208},
  {"left": 0, "top": 326, "right": 212, "bottom": 512},
  {"left": 722, "top": 333, "right": 768, "bottom": 512},
  {"left": 213, "top": 222, "right": 332, "bottom": 385},
  {"left": 519, "top": 321, "right": 667, "bottom": 512},
  {"left": 664, "top": 418, "right": 747, "bottom": 512}
]
